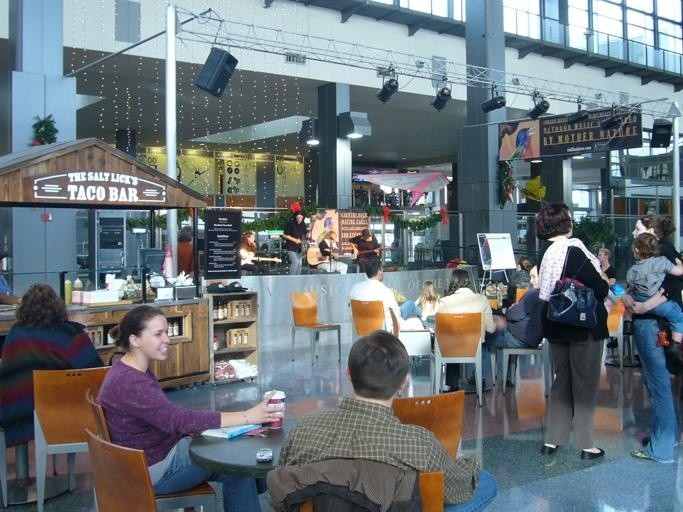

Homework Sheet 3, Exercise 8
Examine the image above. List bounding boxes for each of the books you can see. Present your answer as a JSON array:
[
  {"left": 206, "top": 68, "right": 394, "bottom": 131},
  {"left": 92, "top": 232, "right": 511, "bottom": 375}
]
[{"left": 198, "top": 423, "right": 263, "bottom": 439}]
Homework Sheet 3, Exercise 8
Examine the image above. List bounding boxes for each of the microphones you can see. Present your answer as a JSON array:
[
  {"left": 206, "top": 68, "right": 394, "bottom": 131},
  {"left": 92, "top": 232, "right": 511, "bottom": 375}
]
[
  {"left": 329, "top": 236, "right": 335, "bottom": 241},
  {"left": 254, "top": 240, "right": 259, "bottom": 246}
]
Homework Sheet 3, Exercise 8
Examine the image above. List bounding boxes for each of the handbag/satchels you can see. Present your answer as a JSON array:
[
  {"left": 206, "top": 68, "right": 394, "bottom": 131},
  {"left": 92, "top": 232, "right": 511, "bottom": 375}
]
[{"left": 547, "top": 280, "right": 598, "bottom": 329}]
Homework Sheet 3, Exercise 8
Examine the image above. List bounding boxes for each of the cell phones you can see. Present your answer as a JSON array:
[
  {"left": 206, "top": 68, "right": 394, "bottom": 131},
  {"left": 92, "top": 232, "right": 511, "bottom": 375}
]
[{"left": 256, "top": 448, "right": 272, "bottom": 462}]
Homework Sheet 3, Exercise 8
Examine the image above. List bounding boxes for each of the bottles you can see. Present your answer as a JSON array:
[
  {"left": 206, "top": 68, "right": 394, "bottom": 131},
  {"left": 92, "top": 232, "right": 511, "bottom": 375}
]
[
  {"left": 65, "top": 280, "right": 72, "bottom": 304},
  {"left": 72, "top": 278, "right": 83, "bottom": 291},
  {"left": 167, "top": 322, "right": 180, "bottom": 336},
  {"left": 213, "top": 300, "right": 228, "bottom": 321}
]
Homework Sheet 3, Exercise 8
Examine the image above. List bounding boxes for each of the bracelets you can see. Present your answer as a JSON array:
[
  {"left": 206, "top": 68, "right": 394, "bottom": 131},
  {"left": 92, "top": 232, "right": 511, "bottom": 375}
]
[{"left": 241, "top": 410, "right": 250, "bottom": 426}]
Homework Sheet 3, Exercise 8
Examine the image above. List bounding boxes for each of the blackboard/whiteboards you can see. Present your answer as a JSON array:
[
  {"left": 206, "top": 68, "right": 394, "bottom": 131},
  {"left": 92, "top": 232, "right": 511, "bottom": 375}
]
[{"left": 205, "top": 207, "right": 240, "bottom": 279}]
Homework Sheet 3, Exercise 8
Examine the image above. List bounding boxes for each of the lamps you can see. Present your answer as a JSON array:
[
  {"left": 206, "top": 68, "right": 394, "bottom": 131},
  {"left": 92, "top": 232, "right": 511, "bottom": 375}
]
[
  {"left": 376, "top": 68, "right": 621, "bottom": 131},
  {"left": 338, "top": 111, "right": 372, "bottom": 140},
  {"left": 298, "top": 118, "right": 320, "bottom": 146}
]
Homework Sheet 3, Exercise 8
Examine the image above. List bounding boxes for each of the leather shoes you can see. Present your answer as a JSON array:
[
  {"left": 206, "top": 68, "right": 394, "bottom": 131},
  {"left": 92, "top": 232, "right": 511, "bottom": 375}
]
[
  {"left": 622, "top": 320, "right": 632, "bottom": 334},
  {"left": 542, "top": 443, "right": 559, "bottom": 454},
  {"left": 668, "top": 339, "right": 683, "bottom": 363},
  {"left": 581, "top": 447, "right": 605, "bottom": 460}
]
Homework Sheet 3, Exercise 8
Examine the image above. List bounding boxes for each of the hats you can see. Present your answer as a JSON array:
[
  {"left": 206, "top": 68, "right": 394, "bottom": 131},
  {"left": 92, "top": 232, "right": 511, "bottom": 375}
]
[
  {"left": 225, "top": 282, "right": 248, "bottom": 292},
  {"left": 207, "top": 282, "right": 230, "bottom": 292}
]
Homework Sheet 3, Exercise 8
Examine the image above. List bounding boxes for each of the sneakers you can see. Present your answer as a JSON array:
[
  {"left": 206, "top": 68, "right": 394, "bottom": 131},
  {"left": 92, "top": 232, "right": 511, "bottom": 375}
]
[
  {"left": 642, "top": 436, "right": 651, "bottom": 446},
  {"left": 502, "top": 379, "right": 515, "bottom": 387},
  {"left": 439, "top": 385, "right": 459, "bottom": 394},
  {"left": 468, "top": 376, "right": 485, "bottom": 389}
]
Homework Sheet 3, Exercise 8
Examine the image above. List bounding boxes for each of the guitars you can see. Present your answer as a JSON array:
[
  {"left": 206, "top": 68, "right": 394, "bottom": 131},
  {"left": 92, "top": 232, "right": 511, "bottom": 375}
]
[
  {"left": 240, "top": 249, "right": 284, "bottom": 266},
  {"left": 345, "top": 245, "right": 394, "bottom": 260},
  {"left": 306, "top": 247, "right": 354, "bottom": 264}
]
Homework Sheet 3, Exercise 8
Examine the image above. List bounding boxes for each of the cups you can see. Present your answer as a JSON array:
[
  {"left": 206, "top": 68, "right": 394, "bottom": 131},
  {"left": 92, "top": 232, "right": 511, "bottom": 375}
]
[{"left": 264, "top": 390, "right": 286, "bottom": 431}]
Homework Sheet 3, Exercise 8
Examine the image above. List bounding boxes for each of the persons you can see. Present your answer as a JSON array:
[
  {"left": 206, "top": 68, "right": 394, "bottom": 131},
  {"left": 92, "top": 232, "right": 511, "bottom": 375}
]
[
  {"left": 96, "top": 306, "right": 284, "bottom": 511},
  {"left": 530, "top": 201, "right": 611, "bottom": 462},
  {"left": 315, "top": 231, "right": 348, "bottom": 275},
  {"left": 275, "top": 331, "right": 496, "bottom": 512},
  {"left": 594, "top": 248, "right": 616, "bottom": 289},
  {"left": 176, "top": 225, "right": 202, "bottom": 274},
  {"left": 620, "top": 215, "right": 683, "bottom": 463},
  {"left": 622, "top": 231, "right": 682, "bottom": 362},
  {"left": 349, "top": 228, "right": 383, "bottom": 273},
  {"left": 239, "top": 231, "right": 268, "bottom": 276},
  {"left": 0, "top": 248, "right": 22, "bottom": 306},
  {"left": 282, "top": 211, "right": 312, "bottom": 275},
  {"left": 349, "top": 258, "right": 550, "bottom": 392},
  {"left": 0, "top": 283, "right": 106, "bottom": 446}
]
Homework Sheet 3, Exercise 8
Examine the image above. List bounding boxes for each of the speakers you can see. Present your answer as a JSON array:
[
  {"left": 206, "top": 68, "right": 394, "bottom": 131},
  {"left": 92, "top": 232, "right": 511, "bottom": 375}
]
[
  {"left": 650, "top": 119, "right": 673, "bottom": 148},
  {"left": 196, "top": 47, "right": 238, "bottom": 97}
]
